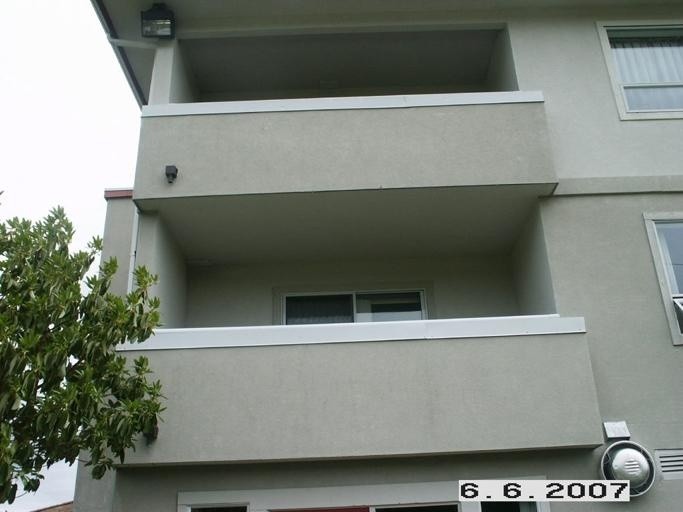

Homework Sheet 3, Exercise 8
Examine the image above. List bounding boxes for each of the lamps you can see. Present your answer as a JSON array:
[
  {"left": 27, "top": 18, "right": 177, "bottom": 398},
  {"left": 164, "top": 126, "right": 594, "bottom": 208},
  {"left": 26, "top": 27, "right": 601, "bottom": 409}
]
[
  {"left": 598, "top": 441, "right": 656, "bottom": 499},
  {"left": 138, "top": 3, "right": 175, "bottom": 39}
]
